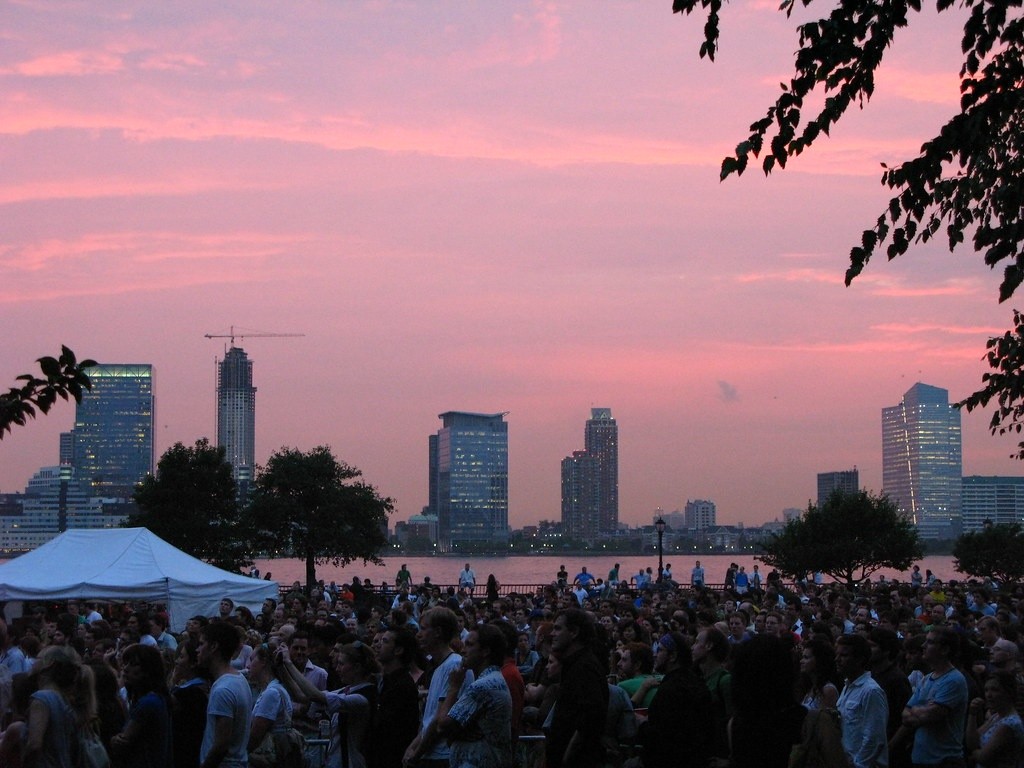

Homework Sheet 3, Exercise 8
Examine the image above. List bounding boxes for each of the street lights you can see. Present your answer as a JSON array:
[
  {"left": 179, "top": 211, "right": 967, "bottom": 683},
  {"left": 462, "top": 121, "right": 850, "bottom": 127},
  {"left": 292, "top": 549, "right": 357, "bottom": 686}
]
[{"left": 654, "top": 516, "right": 667, "bottom": 584}]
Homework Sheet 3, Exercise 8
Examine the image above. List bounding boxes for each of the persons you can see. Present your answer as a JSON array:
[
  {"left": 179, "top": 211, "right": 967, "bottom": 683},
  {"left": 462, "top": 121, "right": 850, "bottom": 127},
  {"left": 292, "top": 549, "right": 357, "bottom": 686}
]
[
  {"left": 485, "top": 573, "right": 499, "bottom": 600},
  {"left": 573, "top": 567, "right": 596, "bottom": 586},
  {"left": 556, "top": 565, "right": 569, "bottom": 586},
  {"left": 459, "top": 563, "right": 477, "bottom": 598},
  {"left": 396, "top": 564, "right": 412, "bottom": 583},
  {"left": 0, "top": 563, "right": 1024, "bottom": 768},
  {"left": 909, "top": 565, "right": 922, "bottom": 587},
  {"left": 691, "top": 561, "right": 704, "bottom": 586},
  {"left": 607, "top": 563, "right": 621, "bottom": 589},
  {"left": 662, "top": 563, "right": 672, "bottom": 579},
  {"left": 767, "top": 569, "right": 783, "bottom": 589},
  {"left": 925, "top": 569, "right": 936, "bottom": 587},
  {"left": 748, "top": 565, "right": 762, "bottom": 588}
]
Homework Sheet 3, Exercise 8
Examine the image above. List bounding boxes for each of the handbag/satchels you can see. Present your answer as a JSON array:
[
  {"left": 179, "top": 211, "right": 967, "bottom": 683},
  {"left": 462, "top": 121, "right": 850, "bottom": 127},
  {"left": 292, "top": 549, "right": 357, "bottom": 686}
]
[
  {"left": 80, "top": 722, "right": 110, "bottom": 768},
  {"left": 246, "top": 688, "right": 305, "bottom": 768}
]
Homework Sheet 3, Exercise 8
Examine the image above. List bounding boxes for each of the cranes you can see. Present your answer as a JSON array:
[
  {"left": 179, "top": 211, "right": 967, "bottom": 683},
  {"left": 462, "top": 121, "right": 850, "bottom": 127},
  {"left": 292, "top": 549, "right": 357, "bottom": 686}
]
[{"left": 200, "top": 323, "right": 304, "bottom": 348}]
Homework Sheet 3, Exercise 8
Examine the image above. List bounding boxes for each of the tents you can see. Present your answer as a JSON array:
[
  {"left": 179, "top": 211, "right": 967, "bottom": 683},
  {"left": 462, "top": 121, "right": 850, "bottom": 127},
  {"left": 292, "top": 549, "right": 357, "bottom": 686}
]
[{"left": 0, "top": 526, "right": 279, "bottom": 635}]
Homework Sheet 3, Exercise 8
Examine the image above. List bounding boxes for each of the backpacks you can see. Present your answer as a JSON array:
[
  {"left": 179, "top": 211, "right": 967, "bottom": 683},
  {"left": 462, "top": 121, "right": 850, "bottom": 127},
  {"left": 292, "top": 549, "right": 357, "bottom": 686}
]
[{"left": 788, "top": 708, "right": 846, "bottom": 768}]
